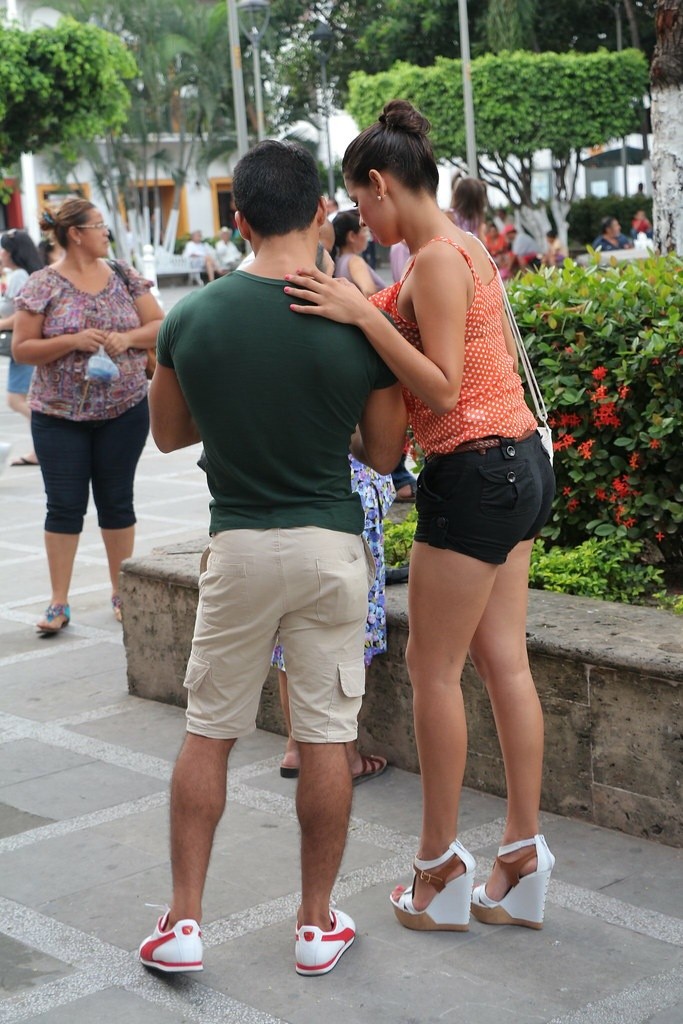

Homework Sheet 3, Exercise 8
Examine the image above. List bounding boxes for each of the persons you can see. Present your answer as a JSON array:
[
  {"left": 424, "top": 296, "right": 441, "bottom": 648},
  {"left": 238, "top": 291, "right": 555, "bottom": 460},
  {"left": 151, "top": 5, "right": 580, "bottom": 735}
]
[
  {"left": 631, "top": 210, "right": 651, "bottom": 238},
  {"left": 0, "top": 228, "right": 44, "bottom": 464},
  {"left": 283, "top": 101, "right": 556, "bottom": 932},
  {"left": 630, "top": 183, "right": 646, "bottom": 199},
  {"left": 316, "top": 199, "right": 409, "bottom": 301},
  {"left": 39, "top": 237, "right": 62, "bottom": 265},
  {"left": 392, "top": 452, "right": 417, "bottom": 502},
  {"left": 484, "top": 209, "right": 566, "bottom": 276},
  {"left": 271, "top": 455, "right": 396, "bottom": 787},
  {"left": 139, "top": 139, "right": 409, "bottom": 978},
  {"left": 185, "top": 231, "right": 228, "bottom": 285},
  {"left": 444, "top": 178, "right": 487, "bottom": 245},
  {"left": 591, "top": 216, "right": 634, "bottom": 251},
  {"left": 212, "top": 226, "right": 244, "bottom": 267},
  {"left": 13, "top": 200, "right": 165, "bottom": 633}
]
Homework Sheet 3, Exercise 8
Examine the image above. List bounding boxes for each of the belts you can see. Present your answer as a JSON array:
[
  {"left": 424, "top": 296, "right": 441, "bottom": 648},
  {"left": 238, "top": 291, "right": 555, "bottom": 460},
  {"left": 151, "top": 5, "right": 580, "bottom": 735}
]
[{"left": 425, "top": 427, "right": 537, "bottom": 465}]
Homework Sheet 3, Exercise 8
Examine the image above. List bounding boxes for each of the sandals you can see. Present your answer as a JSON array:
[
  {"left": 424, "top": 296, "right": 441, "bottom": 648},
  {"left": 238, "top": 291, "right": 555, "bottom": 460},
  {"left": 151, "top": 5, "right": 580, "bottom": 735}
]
[
  {"left": 36, "top": 602, "right": 71, "bottom": 634},
  {"left": 111, "top": 594, "right": 123, "bottom": 624}
]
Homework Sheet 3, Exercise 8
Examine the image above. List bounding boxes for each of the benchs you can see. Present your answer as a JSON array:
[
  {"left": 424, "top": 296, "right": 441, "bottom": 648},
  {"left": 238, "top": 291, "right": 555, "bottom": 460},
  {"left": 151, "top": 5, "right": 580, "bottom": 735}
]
[{"left": 154, "top": 255, "right": 222, "bottom": 286}]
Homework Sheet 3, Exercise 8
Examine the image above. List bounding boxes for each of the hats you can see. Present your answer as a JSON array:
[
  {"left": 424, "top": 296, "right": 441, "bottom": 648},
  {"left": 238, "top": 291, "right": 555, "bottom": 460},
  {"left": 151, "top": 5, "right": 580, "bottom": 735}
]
[{"left": 221, "top": 226, "right": 230, "bottom": 233}]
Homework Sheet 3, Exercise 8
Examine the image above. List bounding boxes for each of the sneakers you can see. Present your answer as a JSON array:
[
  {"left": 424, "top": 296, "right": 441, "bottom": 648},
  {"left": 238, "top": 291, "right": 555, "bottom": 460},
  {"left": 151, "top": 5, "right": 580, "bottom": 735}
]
[
  {"left": 138, "top": 903, "right": 205, "bottom": 972},
  {"left": 294, "top": 908, "right": 357, "bottom": 976}
]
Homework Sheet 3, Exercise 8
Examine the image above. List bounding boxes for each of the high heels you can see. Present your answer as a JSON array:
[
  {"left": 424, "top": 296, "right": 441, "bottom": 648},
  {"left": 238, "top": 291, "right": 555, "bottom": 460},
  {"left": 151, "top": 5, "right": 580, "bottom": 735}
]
[
  {"left": 471, "top": 834, "right": 556, "bottom": 930},
  {"left": 389, "top": 838, "right": 477, "bottom": 932}
]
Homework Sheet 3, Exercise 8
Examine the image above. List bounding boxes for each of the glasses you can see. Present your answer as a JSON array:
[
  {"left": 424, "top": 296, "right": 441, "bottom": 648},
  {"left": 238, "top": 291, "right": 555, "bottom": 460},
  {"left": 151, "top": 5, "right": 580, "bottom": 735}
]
[
  {"left": 6, "top": 228, "right": 18, "bottom": 241},
  {"left": 76, "top": 223, "right": 109, "bottom": 229}
]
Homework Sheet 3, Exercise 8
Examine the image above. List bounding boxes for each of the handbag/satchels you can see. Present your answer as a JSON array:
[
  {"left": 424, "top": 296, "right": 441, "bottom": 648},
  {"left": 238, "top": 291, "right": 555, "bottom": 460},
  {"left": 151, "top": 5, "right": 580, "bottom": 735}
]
[
  {"left": 535, "top": 423, "right": 554, "bottom": 467},
  {"left": 144, "top": 347, "right": 158, "bottom": 378},
  {"left": 0, "top": 330, "right": 14, "bottom": 356}
]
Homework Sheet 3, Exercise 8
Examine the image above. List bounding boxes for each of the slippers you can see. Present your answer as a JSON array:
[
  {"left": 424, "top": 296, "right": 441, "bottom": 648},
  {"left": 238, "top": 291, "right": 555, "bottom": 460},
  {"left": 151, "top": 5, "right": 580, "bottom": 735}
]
[
  {"left": 392, "top": 490, "right": 419, "bottom": 504},
  {"left": 352, "top": 751, "right": 387, "bottom": 786},
  {"left": 279, "top": 765, "right": 301, "bottom": 779}
]
